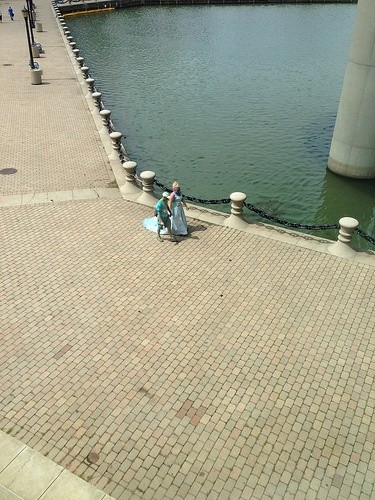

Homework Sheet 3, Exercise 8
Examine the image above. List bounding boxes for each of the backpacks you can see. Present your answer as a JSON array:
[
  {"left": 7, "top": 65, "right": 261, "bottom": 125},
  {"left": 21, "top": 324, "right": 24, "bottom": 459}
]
[{"left": 155, "top": 200, "right": 163, "bottom": 217}]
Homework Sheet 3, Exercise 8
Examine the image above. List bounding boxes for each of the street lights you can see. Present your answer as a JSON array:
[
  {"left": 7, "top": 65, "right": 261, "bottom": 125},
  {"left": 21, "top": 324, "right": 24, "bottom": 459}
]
[{"left": 21, "top": 6, "right": 35, "bottom": 70}]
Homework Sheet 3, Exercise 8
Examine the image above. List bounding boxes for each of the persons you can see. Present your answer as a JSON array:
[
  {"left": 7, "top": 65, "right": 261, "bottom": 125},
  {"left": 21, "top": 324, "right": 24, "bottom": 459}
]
[
  {"left": 0, "top": 11, "right": 3, "bottom": 23},
  {"left": 7, "top": 6, "right": 15, "bottom": 21},
  {"left": 168, "top": 181, "right": 190, "bottom": 236},
  {"left": 154, "top": 191, "right": 182, "bottom": 243}
]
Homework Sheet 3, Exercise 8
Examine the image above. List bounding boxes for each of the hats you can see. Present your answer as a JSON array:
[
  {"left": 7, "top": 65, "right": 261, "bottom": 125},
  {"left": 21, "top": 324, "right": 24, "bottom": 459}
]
[{"left": 162, "top": 192, "right": 171, "bottom": 199}]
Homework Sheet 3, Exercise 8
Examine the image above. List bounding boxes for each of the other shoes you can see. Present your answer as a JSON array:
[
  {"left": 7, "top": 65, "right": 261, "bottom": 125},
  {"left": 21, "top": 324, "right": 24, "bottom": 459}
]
[
  {"left": 158, "top": 237, "right": 164, "bottom": 242},
  {"left": 171, "top": 238, "right": 178, "bottom": 242}
]
